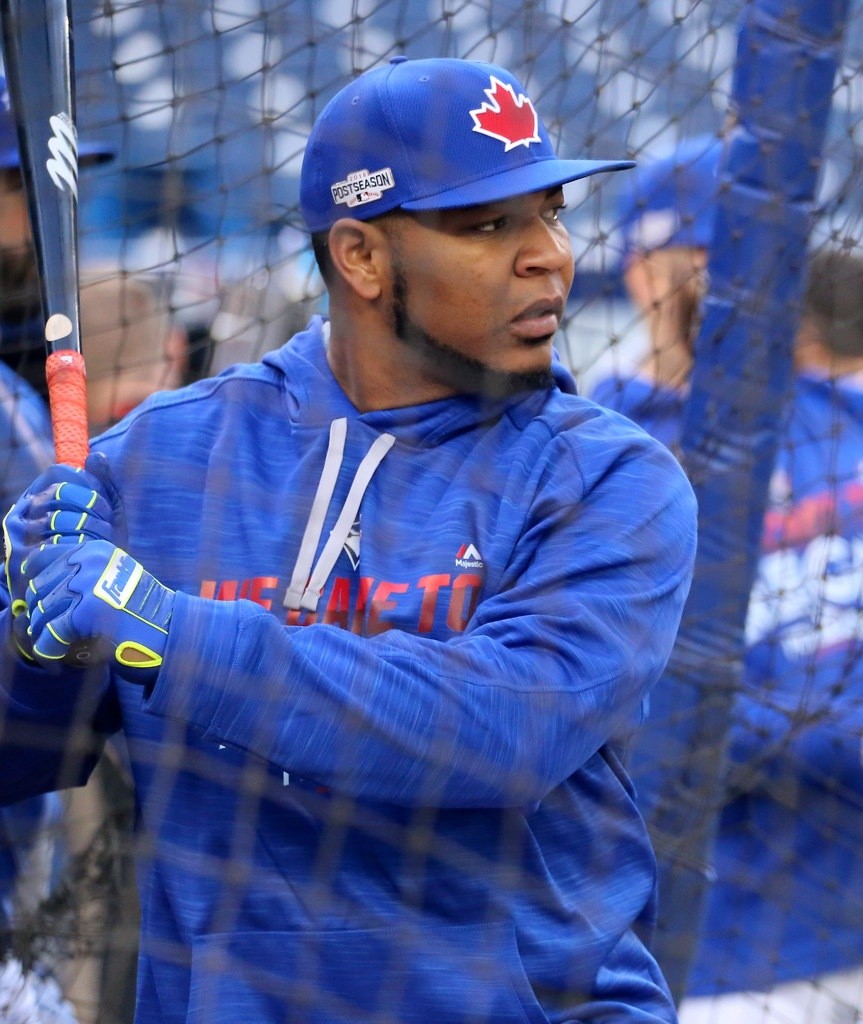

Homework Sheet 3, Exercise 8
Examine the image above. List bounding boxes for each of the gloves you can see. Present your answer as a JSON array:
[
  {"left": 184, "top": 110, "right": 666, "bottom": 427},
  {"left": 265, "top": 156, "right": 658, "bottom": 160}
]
[
  {"left": 14, "top": 539, "right": 177, "bottom": 679},
  {"left": 3, "top": 452, "right": 126, "bottom": 605}
]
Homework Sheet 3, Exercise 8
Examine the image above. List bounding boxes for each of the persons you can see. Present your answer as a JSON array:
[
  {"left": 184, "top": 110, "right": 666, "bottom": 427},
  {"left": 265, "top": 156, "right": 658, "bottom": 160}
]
[
  {"left": 0, "top": 56, "right": 699, "bottom": 1024},
  {"left": 795, "top": 251, "right": 863, "bottom": 397},
  {"left": 0, "top": 75, "right": 115, "bottom": 974},
  {"left": 578, "top": 138, "right": 863, "bottom": 1000}
]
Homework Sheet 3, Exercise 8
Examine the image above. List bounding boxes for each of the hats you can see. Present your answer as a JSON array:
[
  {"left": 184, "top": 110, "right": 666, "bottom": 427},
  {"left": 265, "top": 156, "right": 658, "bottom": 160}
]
[
  {"left": 300, "top": 55, "right": 639, "bottom": 234},
  {"left": 0, "top": 57, "right": 117, "bottom": 178},
  {"left": 609, "top": 118, "right": 771, "bottom": 277}
]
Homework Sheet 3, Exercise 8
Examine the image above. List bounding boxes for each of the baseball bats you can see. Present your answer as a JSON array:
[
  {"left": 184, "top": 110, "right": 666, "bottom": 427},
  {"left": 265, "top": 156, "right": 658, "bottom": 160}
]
[{"left": 0, "top": 0, "right": 99, "bottom": 483}]
